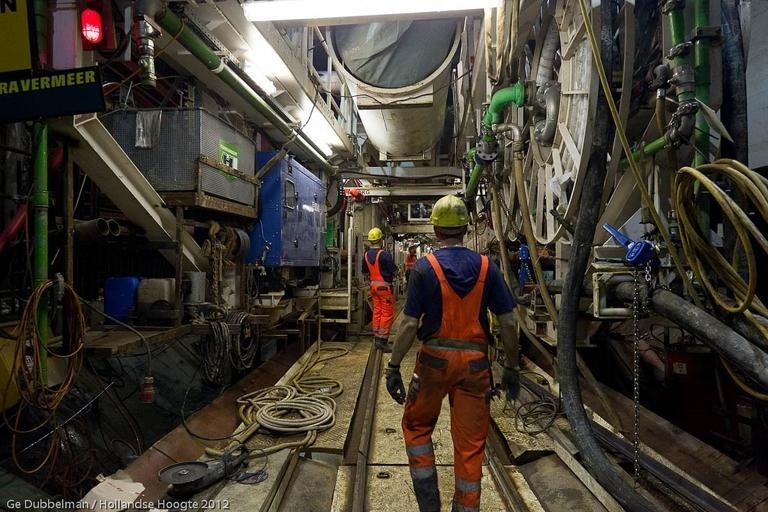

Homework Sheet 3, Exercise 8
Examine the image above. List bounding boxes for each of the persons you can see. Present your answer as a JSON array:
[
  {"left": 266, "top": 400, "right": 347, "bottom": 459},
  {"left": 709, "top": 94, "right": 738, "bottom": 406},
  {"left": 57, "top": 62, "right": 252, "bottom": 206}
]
[
  {"left": 383, "top": 193, "right": 524, "bottom": 512},
  {"left": 403, "top": 244, "right": 418, "bottom": 281},
  {"left": 361, "top": 227, "right": 397, "bottom": 355}
]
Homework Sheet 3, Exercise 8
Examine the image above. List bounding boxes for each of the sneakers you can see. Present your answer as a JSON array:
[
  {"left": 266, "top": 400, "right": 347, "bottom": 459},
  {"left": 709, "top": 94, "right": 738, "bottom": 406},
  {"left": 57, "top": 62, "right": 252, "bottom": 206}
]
[{"left": 373, "top": 335, "right": 393, "bottom": 353}]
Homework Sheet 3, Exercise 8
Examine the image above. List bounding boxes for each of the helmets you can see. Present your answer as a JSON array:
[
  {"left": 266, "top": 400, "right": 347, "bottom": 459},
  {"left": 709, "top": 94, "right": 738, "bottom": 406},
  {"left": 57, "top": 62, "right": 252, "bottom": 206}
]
[
  {"left": 367, "top": 227, "right": 384, "bottom": 242},
  {"left": 428, "top": 193, "right": 470, "bottom": 228}
]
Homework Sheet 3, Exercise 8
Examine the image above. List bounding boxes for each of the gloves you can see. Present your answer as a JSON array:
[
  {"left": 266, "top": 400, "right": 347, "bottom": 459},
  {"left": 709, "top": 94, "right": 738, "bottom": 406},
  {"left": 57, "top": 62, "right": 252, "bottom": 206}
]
[
  {"left": 383, "top": 367, "right": 407, "bottom": 406},
  {"left": 498, "top": 366, "right": 521, "bottom": 402}
]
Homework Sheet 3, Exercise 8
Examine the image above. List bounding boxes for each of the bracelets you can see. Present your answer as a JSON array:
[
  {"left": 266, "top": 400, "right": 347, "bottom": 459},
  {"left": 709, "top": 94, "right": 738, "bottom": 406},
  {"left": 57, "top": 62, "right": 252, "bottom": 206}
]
[{"left": 388, "top": 361, "right": 400, "bottom": 368}]
